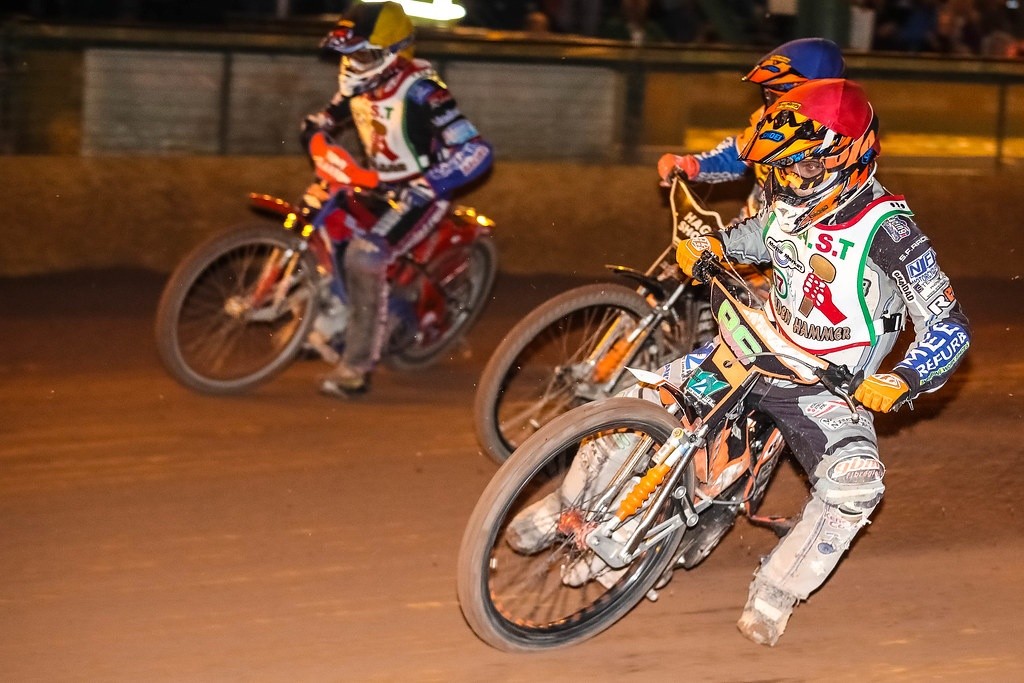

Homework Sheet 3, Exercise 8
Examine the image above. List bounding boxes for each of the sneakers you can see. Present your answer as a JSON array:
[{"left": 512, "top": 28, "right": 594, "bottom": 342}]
[
  {"left": 505, "top": 491, "right": 568, "bottom": 555},
  {"left": 736, "top": 575, "right": 795, "bottom": 645},
  {"left": 316, "top": 369, "right": 370, "bottom": 400}
]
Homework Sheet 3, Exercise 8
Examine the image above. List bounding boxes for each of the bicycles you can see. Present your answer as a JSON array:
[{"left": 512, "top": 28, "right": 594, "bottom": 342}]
[
  {"left": 455, "top": 248, "right": 906, "bottom": 655},
  {"left": 471, "top": 166, "right": 769, "bottom": 482},
  {"left": 155, "top": 125, "right": 502, "bottom": 399}
]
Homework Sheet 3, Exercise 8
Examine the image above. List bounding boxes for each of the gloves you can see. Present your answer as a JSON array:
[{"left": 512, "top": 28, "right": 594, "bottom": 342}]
[
  {"left": 656, "top": 152, "right": 699, "bottom": 188},
  {"left": 854, "top": 371, "right": 910, "bottom": 413},
  {"left": 675, "top": 235, "right": 726, "bottom": 278},
  {"left": 301, "top": 111, "right": 341, "bottom": 139},
  {"left": 387, "top": 178, "right": 435, "bottom": 207}
]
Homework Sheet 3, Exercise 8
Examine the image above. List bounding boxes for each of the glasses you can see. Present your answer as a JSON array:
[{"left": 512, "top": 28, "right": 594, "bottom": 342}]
[
  {"left": 774, "top": 156, "right": 830, "bottom": 192},
  {"left": 340, "top": 45, "right": 389, "bottom": 80}
]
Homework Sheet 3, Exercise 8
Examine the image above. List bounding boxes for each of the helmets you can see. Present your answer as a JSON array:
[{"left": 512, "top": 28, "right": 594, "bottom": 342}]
[
  {"left": 742, "top": 38, "right": 846, "bottom": 111},
  {"left": 739, "top": 79, "right": 880, "bottom": 236},
  {"left": 316, "top": 1, "right": 416, "bottom": 96}
]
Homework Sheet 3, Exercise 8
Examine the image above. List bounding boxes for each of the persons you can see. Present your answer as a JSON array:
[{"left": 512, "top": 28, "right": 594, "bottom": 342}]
[
  {"left": 561, "top": 36, "right": 847, "bottom": 404},
  {"left": 301, "top": 0, "right": 494, "bottom": 396},
  {"left": 506, "top": 77, "right": 971, "bottom": 647},
  {"left": 514, "top": 0, "right": 1023, "bottom": 59}
]
